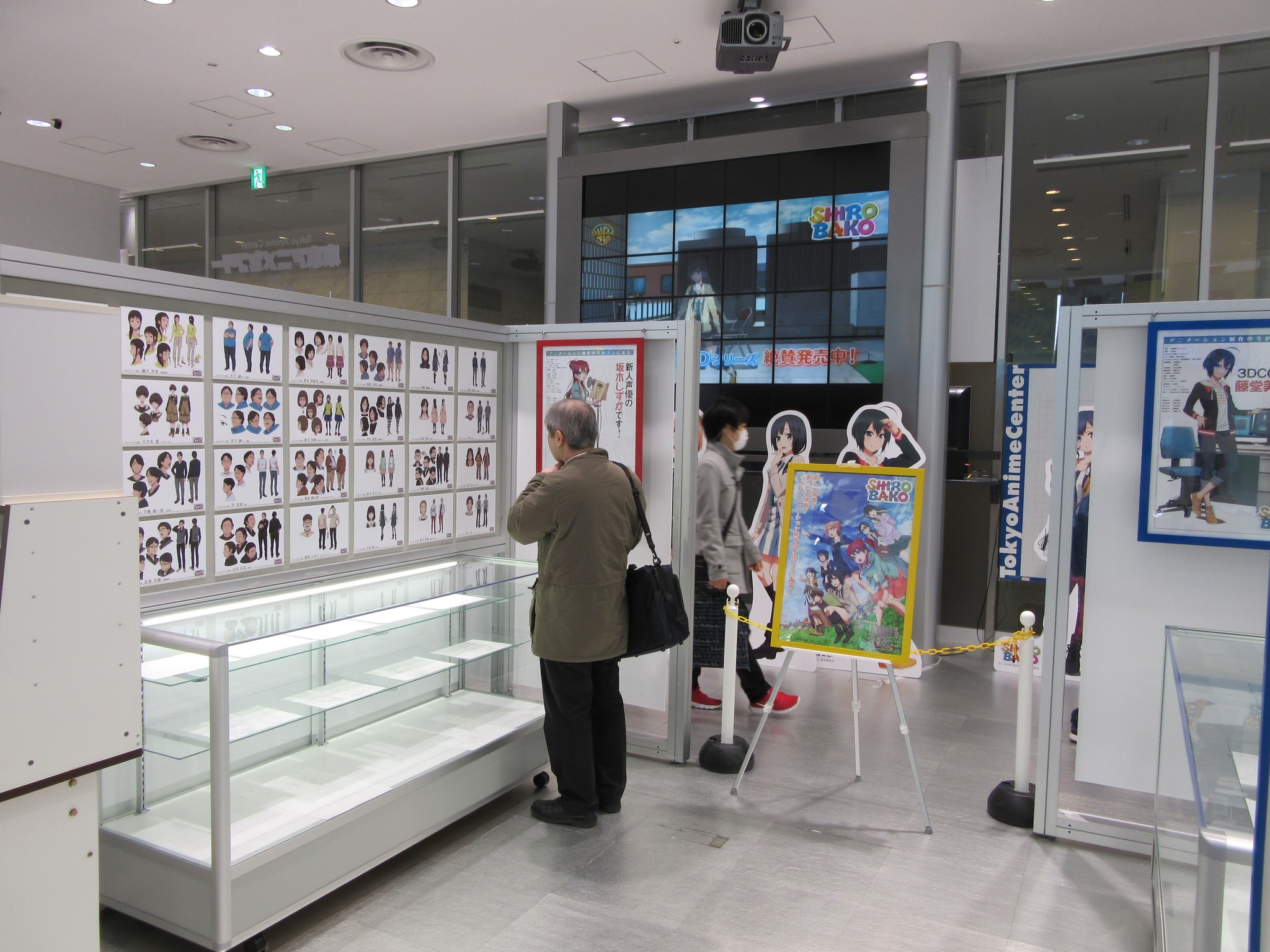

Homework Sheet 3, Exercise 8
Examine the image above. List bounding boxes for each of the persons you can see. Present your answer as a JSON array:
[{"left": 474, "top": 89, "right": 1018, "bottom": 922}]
[
  {"left": 507, "top": 399, "right": 648, "bottom": 828},
  {"left": 671, "top": 395, "right": 800, "bottom": 718}
]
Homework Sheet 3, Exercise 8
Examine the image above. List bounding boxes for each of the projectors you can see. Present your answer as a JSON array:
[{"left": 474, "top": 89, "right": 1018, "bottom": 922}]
[{"left": 715, "top": 8, "right": 791, "bottom": 76}]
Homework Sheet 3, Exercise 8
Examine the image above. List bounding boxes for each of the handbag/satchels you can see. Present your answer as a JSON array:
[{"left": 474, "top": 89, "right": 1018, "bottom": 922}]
[
  {"left": 693, "top": 580, "right": 752, "bottom": 671},
  {"left": 691, "top": 555, "right": 708, "bottom": 581},
  {"left": 624, "top": 564, "right": 690, "bottom": 657}
]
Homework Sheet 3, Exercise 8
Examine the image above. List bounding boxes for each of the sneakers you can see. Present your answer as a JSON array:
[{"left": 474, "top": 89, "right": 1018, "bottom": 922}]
[
  {"left": 748, "top": 686, "right": 801, "bottom": 714},
  {"left": 690, "top": 686, "right": 722, "bottom": 710}
]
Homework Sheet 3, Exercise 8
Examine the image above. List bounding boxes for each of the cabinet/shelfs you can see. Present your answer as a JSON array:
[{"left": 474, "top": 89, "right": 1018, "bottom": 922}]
[
  {"left": 95, "top": 554, "right": 541, "bottom": 952},
  {"left": 1147, "top": 619, "right": 1266, "bottom": 952}
]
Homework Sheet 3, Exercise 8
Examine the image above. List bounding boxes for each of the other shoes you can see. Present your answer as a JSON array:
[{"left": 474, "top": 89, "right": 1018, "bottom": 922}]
[
  {"left": 531, "top": 796, "right": 597, "bottom": 828},
  {"left": 597, "top": 796, "right": 622, "bottom": 813},
  {"left": 1068, "top": 707, "right": 1080, "bottom": 741}
]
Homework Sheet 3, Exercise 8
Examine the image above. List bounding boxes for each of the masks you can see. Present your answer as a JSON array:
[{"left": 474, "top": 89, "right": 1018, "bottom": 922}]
[{"left": 723, "top": 424, "right": 749, "bottom": 451}]
